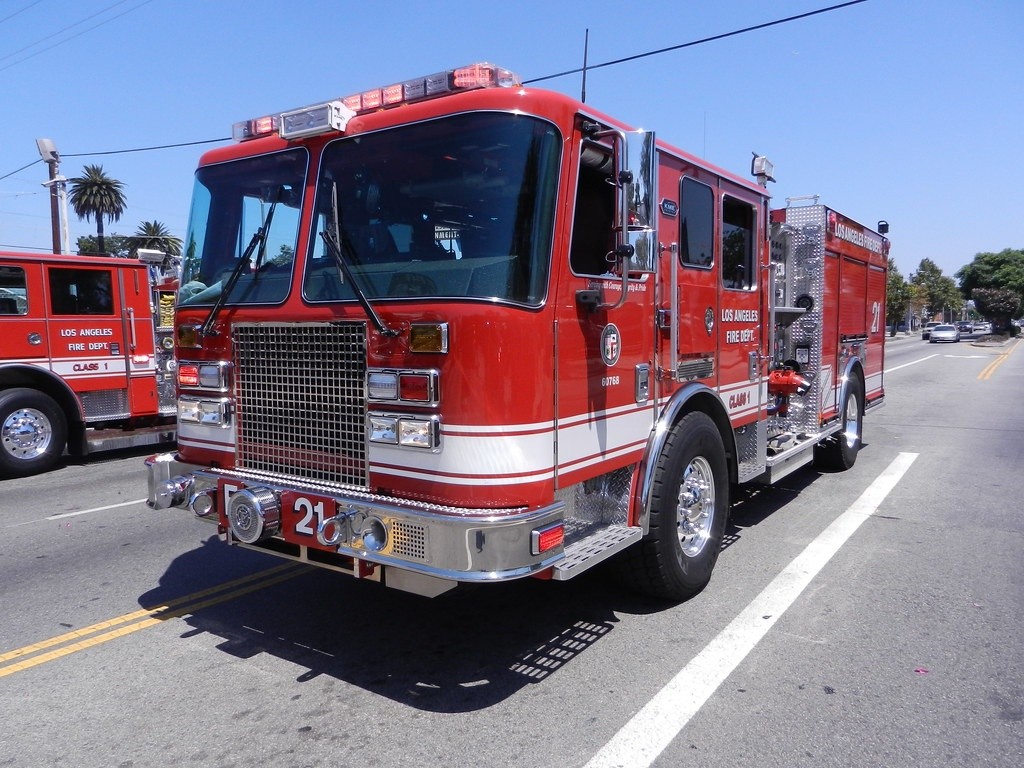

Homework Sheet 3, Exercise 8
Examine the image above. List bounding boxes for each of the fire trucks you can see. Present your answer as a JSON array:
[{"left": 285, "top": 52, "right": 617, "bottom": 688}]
[
  {"left": 146, "top": 61, "right": 891, "bottom": 599},
  {"left": 0, "top": 245, "right": 183, "bottom": 479}
]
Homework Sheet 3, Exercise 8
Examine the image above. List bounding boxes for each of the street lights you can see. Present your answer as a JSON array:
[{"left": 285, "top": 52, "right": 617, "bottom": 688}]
[
  {"left": 908, "top": 270, "right": 926, "bottom": 335},
  {"left": 41, "top": 175, "right": 70, "bottom": 255},
  {"left": 36, "top": 137, "right": 62, "bottom": 255}
]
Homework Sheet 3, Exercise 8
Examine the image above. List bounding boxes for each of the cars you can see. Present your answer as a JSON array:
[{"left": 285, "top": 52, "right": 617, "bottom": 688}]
[
  {"left": 1011, "top": 319, "right": 1024, "bottom": 327},
  {"left": 973, "top": 323, "right": 986, "bottom": 331},
  {"left": 929, "top": 325, "right": 961, "bottom": 342},
  {"left": 982, "top": 322, "right": 992, "bottom": 329}
]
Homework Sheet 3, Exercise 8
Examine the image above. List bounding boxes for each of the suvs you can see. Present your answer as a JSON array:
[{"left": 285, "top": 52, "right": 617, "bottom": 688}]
[
  {"left": 922, "top": 322, "right": 943, "bottom": 339},
  {"left": 957, "top": 322, "right": 973, "bottom": 333}
]
